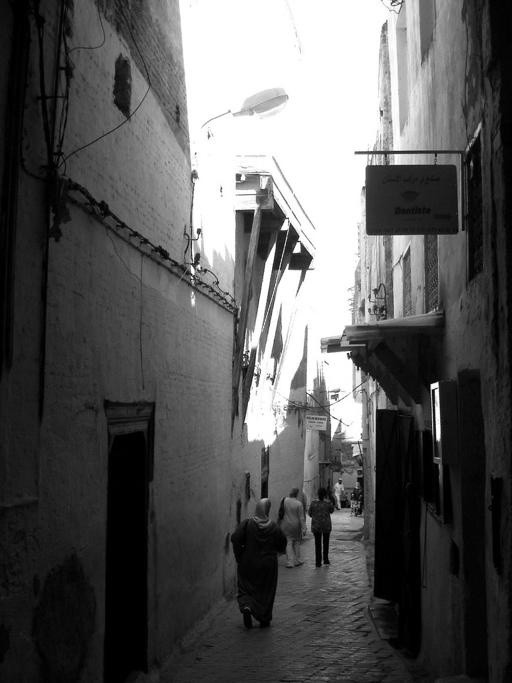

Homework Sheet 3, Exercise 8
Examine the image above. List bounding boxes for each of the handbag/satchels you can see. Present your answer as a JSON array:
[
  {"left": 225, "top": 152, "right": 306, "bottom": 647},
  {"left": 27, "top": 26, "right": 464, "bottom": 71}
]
[{"left": 279, "top": 497, "right": 287, "bottom": 519}]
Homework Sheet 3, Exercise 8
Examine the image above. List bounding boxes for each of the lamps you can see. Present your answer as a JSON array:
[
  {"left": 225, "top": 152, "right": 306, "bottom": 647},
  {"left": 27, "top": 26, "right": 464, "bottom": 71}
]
[{"left": 367, "top": 283, "right": 388, "bottom": 322}]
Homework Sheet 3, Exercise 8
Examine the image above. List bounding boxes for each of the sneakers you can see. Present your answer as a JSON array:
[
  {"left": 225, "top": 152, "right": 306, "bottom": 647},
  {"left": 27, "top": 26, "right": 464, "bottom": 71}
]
[
  {"left": 244, "top": 607, "right": 252, "bottom": 629},
  {"left": 286, "top": 561, "right": 304, "bottom": 568},
  {"left": 260, "top": 622, "right": 270, "bottom": 627}
]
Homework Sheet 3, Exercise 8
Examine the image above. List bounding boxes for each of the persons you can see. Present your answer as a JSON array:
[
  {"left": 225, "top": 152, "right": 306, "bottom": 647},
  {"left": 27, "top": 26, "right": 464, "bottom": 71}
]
[
  {"left": 350, "top": 482, "right": 364, "bottom": 514},
  {"left": 334, "top": 478, "right": 345, "bottom": 510},
  {"left": 276, "top": 488, "right": 307, "bottom": 568},
  {"left": 309, "top": 487, "right": 334, "bottom": 567},
  {"left": 231, "top": 498, "right": 288, "bottom": 629}
]
